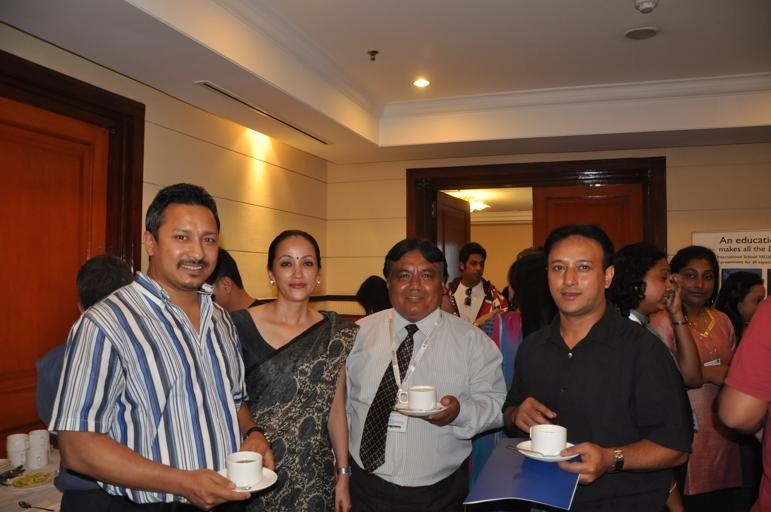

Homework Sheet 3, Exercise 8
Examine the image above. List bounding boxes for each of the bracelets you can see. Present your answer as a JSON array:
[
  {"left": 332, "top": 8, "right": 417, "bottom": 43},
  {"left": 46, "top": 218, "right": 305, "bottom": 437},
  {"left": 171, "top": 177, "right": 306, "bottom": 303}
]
[
  {"left": 472, "top": 321, "right": 480, "bottom": 328},
  {"left": 673, "top": 315, "right": 689, "bottom": 325},
  {"left": 244, "top": 427, "right": 264, "bottom": 440},
  {"left": 337, "top": 466, "right": 352, "bottom": 476},
  {"left": 614, "top": 449, "right": 624, "bottom": 472}
]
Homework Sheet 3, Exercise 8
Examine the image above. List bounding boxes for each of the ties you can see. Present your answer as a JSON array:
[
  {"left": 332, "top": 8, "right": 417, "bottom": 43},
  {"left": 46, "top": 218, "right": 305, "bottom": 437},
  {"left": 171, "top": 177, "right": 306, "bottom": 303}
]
[{"left": 359, "top": 323, "right": 420, "bottom": 473}]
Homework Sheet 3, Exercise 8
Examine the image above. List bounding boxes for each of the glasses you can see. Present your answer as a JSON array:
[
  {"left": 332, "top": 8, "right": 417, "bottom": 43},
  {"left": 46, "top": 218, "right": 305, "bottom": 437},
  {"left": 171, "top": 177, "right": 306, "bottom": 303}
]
[{"left": 464, "top": 286, "right": 472, "bottom": 307}]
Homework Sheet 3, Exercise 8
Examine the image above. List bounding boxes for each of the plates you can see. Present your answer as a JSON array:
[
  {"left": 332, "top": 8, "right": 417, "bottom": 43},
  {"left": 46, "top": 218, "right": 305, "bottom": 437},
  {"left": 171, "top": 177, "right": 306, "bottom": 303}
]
[
  {"left": 515, "top": 440, "right": 580, "bottom": 463},
  {"left": 11, "top": 475, "right": 52, "bottom": 489},
  {"left": 393, "top": 402, "right": 447, "bottom": 416},
  {"left": 217, "top": 465, "right": 278, "bottom": 492}
]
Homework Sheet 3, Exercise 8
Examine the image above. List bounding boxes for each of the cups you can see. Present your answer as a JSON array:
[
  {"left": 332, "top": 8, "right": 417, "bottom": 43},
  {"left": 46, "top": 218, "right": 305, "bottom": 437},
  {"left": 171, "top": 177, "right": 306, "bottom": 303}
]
[
  {"left": 530, "top": 424, "right": 567, "bottom": 455},
  {"left": 398, "top": 385, "right": 436, "bottom": 410},
  {"left": 226, "top": 450, "right": 263, "bottom": 487},
  {"left": 8, "top": 429, "right": 50, "bottom": 468}
]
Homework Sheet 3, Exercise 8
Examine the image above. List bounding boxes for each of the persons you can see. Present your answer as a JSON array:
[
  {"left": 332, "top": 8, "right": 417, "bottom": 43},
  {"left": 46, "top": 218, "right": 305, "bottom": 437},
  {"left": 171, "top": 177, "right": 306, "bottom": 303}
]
[
  {"left": 714, "top": 271, "right": 765, "bottom": 345},
  {"left": 205, "top": 246, "right": 277, "bottom": 313},
  {"left": 34, "top": 253, "right": 135, "bottom": 512},
  {"left": 47, "top": 184, "right": 274, "bottom": 512},
  {"left": 716, "top": 297, "right": 771, "bottom": 512},
  {"left": 500, "top": 225, "right": 695, "bottom": 512},
  {"left": 646, "top": 246, "right": 745, "bottom": 512},
  {"left": 609, "top": 242, "right": 705, "bottom": 390},
  {"left": 449, "top": 243, "right": 508, "bottom": 324},
  {"left": 344, "top": 238, "right": 509, "bottom": 512},
  {"left": 468, "top": 246, "right": 558, "bottom": 489},
  {"left": 356, "top": 276, "right": 392, "bottom": 315},
  {"left": 229, "top": 229, "right": 353, "bottom": 512}
]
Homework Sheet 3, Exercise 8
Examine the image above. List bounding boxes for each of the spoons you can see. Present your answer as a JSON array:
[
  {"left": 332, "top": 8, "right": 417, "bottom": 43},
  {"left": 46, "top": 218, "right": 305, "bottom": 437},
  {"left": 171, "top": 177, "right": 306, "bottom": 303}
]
[{"left": 18, "top": 500, "right": 55, "bottom": 512}]
